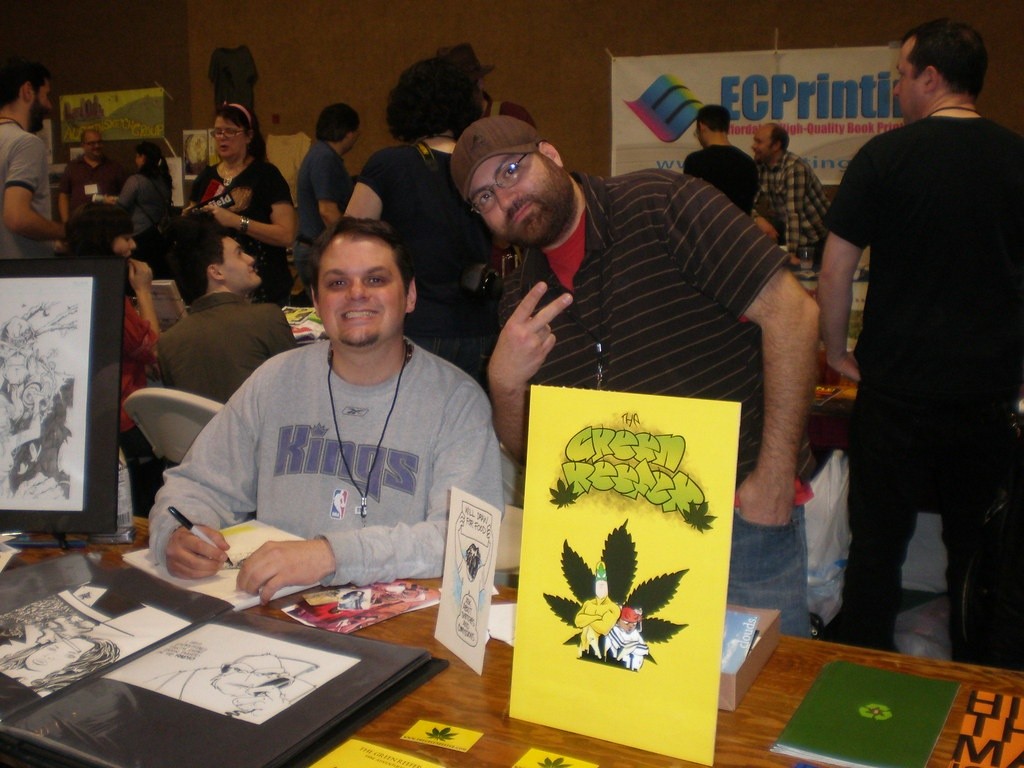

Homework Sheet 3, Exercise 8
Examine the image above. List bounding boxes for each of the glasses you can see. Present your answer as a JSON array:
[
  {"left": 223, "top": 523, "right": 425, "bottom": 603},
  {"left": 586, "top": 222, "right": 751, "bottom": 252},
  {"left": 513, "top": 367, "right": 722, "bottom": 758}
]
[
  {"left": 210, "top": 129, "right": 244, "bottom": 137},
  {"left": 84, "top": 140, "right": 104, "bottom": 146},
  {"left": 470, "top": 152, "right": 529, "bottom": 214}
]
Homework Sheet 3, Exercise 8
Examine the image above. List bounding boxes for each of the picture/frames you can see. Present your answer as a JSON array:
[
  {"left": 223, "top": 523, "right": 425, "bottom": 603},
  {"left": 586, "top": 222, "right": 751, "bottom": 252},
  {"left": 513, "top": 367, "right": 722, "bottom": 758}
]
[{"left": 0, "top": 260, "right": 132, "bottom": 533}]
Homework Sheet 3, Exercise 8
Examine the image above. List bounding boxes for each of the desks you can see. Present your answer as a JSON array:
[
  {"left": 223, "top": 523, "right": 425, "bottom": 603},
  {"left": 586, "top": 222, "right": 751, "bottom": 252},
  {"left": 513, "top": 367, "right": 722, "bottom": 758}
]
[{"left": 0, "top": 516, "right": 1024, "bottom": 768}]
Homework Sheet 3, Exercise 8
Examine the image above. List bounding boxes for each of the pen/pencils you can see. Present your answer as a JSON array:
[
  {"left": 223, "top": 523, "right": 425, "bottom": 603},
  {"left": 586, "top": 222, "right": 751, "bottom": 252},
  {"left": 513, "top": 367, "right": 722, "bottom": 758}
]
[
  {"left": 4, "top": 539, "right": 85, "bottom": 548},
  {"left": 166, "top": 506, "right": 235, "bottom": 570}
]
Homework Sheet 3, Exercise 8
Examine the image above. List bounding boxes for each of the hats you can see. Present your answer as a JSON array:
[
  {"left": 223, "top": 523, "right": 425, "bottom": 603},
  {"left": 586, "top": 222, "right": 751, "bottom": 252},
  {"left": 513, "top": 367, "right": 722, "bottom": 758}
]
[
  {"left": 436, "top": 42, "right": 495, "bottom": 80},
  {"left": 450, "top": 114, "right": 546, "bottom": 202}
]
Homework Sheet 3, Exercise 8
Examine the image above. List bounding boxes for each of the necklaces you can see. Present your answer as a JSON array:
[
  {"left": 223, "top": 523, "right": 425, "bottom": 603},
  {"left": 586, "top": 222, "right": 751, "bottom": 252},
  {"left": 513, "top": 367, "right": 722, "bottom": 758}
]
[
  {"left": 926, "top": 106, "right": 982, "bottom": 119},
  {"left": 328, "top": 339, "right": 413, "bottom": 517}
]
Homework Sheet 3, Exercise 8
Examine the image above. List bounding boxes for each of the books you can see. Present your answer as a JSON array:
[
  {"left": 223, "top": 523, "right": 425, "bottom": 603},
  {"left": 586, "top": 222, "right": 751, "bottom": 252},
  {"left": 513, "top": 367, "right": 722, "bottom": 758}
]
[{"left": 770, "top": 661, "right": 961, "bottom": 768}]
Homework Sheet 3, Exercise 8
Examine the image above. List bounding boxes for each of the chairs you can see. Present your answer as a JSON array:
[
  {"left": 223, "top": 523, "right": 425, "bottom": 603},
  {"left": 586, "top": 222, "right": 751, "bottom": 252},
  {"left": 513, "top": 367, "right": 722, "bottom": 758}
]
[{"left": 123, "top": 387, "right": 223, "bottom": 465}]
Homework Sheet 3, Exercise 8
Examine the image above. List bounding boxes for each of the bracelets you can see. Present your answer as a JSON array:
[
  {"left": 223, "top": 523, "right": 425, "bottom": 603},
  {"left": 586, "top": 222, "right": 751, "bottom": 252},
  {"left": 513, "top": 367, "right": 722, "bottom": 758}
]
[{"left": 240, "top": 215, "right": 249, "bottom": 235}]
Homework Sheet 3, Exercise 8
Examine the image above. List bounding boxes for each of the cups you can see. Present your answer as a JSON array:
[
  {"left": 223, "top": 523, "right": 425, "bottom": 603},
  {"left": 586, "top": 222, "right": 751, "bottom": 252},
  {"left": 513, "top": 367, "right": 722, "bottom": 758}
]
[{"left": 799, "top": 248, "right": 814, "bottom": 268}]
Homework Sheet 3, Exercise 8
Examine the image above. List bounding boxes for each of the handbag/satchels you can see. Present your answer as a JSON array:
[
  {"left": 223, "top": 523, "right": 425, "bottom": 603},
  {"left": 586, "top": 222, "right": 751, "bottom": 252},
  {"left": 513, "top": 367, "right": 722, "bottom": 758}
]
[{"left": 805, "top": 449, "right": 852, "bottom": 630}]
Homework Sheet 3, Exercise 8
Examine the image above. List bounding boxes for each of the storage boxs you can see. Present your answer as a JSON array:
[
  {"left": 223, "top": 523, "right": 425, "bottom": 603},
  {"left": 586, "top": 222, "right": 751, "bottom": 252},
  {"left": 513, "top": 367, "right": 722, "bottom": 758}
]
[{"left": 719, "top": 602, "right": 781, "bottom": 712}]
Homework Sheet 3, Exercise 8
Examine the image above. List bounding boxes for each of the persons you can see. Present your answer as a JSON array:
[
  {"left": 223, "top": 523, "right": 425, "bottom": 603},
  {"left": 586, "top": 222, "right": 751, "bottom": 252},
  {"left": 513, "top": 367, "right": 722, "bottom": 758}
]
[
  {"left": 683, "top": 104, "right": 832, "bottom": 273},
  {"left": 819, "top": 19, "right": 1024, "bottom": 669},
  {"left": 330, "top": 42, "right": 540, "bottom": 408},
  {"left": 65, "top": 201, "right": 321, "bottom": 511},
  {"left": 449, "top": 115, "right": 818, "bottom": 635},
  {"left": 0, "top": 60, "right": 359, "bottom": 304},
  {"left": 149, "top": 215, "right": 506, "bottom": 606}
]
